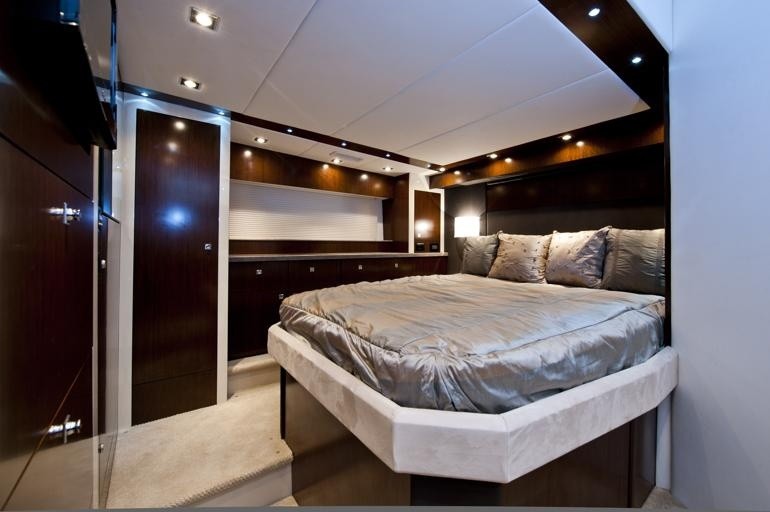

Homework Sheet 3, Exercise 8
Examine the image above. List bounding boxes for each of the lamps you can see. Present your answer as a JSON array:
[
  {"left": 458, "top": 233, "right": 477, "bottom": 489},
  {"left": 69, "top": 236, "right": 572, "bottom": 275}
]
[{"left": 453, "top": 214, "right": 482, "bottom": 240}]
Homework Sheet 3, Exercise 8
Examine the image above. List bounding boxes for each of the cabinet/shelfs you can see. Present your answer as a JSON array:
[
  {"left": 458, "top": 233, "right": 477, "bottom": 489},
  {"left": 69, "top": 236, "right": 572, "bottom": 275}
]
[
  {"left": 230, "top": 141, "right": 395, "bottom": 200},
  {"left": 1, "top": 1, "right": 124, "bottom": 511},
  {"left": 340, "top": 258, "right": 446, "bottom": 285},
  {"left": 229, "top": 259, "right": 340, "bottom": 360}
]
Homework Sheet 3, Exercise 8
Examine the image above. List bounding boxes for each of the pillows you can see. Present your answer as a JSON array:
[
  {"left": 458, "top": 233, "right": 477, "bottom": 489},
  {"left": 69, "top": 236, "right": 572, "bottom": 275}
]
[
  {"left": 461, "top": 229, "right": 504, "bottom": 275},
  {"left": 544, "top": 224, "right": 607, "bottom": 289},
  {"left": 484, "top": 230, "right": 556, "bottom": 283},
  {"left": 599, "top": 226, "right": 667, "bottom": 296}
]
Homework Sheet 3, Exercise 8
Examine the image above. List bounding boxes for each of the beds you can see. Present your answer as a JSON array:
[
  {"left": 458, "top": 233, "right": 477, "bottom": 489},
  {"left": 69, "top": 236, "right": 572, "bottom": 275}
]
[{"left": 263, "top": 225, "right": 674, "bottom": 508}]
